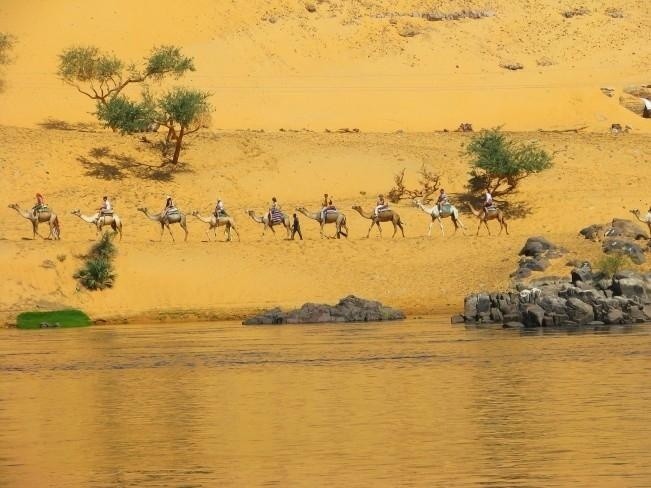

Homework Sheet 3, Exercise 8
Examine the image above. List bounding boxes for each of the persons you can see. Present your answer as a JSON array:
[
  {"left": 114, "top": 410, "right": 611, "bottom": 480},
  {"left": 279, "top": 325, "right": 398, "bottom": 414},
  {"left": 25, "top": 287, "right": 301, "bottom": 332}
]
[
  {"left": 373, "top": 193, "right": 387, "bottom": 218},
  {"left": 162, "top": 194, "right": 174, "bottom": 218},
  {"left": 215, "top": 198, "right": 225, "bottom": 220},
  {"left": 291, "top": 213, "right": 303, "bottom": 240},
  {"left": 99, "top": 196, "right": 111, "bottom": 217},
  {"left": 33, "top": 192, "right": 44, "bottom": 216},
  {"left": 321, "top": 193, "right": 336, "bottom": 226},
  {"left": 483, "top": 187, "right": 493, "bottom": 222},
  {"left": 437, "top": 188, "right": 448, "bottom": 213},
  {"left": 269, "top": 197, "right": 278, "bottom": 221}
]
